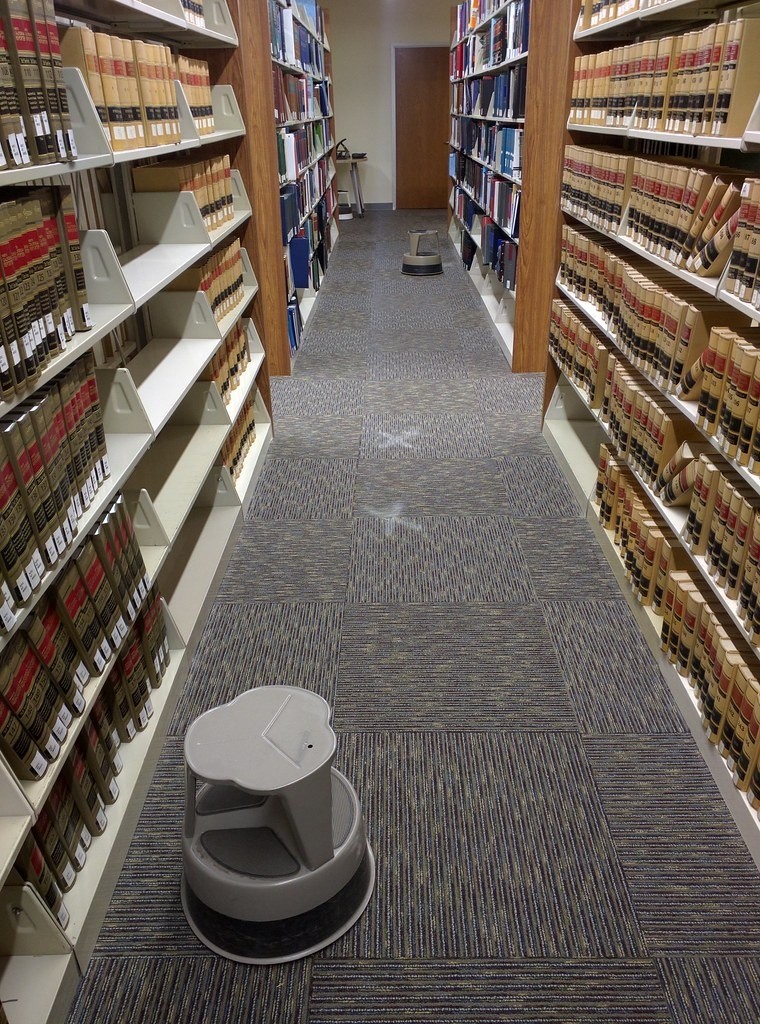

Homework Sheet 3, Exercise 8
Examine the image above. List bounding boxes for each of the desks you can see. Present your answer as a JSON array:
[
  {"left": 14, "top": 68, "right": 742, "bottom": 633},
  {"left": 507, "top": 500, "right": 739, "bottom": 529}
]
[{"left": 337, "top": 156, "right": 368, "bottom": 219}]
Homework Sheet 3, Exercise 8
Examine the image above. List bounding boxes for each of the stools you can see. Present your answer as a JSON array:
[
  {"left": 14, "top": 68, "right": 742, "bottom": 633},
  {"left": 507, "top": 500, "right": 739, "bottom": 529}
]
[
  {"left": 337, "top": 189, "right": 354, "bottom": 220},
  {"left": 179, "top": 680, "right": 377, "bottom": 967},
  {"left": 401, "top": 229, "right": 444, "bottom": 276}
]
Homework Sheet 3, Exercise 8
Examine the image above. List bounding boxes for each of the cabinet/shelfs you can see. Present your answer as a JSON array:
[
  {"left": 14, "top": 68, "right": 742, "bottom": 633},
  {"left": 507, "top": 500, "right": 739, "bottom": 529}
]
[{"left": 1, "top": 0, "right": 760, "bottom": 1024}]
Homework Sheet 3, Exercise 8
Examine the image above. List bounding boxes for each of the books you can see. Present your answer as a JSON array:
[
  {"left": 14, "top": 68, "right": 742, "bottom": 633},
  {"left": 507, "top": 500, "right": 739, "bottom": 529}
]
[{"left": 1, "top": 0, "right": 760, "bottom": 930}]
